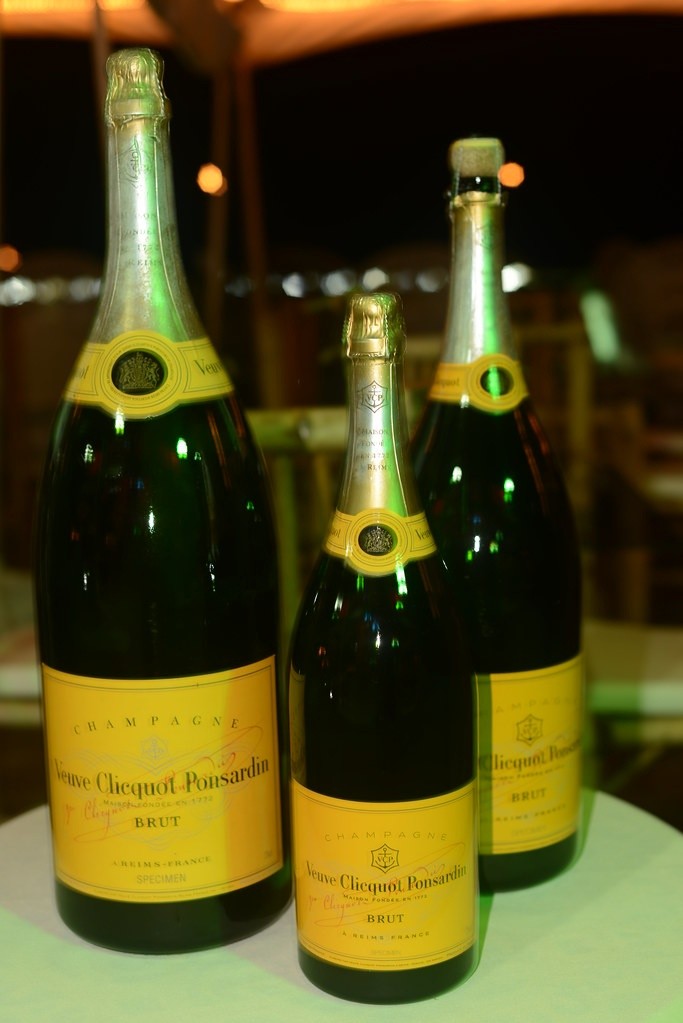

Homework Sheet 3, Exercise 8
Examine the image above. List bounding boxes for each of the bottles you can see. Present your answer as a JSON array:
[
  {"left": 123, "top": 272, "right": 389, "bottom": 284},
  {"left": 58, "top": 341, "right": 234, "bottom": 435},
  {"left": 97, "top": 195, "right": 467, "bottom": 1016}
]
[
  {"left": 32, "top": 46, "right": 295, "bottom": 955},
  {"left": 408, "top": 138, "right": 583, "bottom": 896},
  {"left": 291, "top": 289, "right": 482, "bottom": 1006}
]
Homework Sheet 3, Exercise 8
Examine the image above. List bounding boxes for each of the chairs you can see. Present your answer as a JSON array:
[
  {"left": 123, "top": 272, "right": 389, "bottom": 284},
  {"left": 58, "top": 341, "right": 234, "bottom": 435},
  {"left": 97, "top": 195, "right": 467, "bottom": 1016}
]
[{"left": 232, "top": 312, "right": 681, "bottom": 794}]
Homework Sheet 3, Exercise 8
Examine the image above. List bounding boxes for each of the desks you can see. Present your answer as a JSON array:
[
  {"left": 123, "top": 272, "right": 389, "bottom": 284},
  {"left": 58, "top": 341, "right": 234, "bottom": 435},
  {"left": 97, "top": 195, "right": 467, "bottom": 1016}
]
[{"left": 1, "top": 784, "right": 683, "bottom": 1023}]
